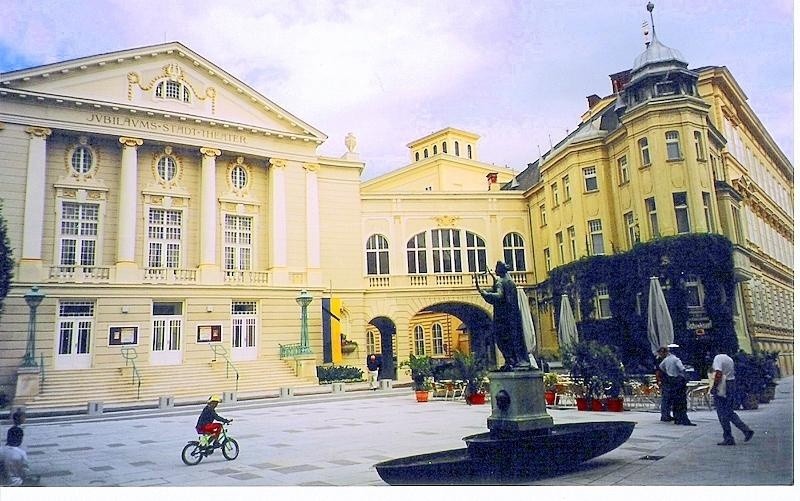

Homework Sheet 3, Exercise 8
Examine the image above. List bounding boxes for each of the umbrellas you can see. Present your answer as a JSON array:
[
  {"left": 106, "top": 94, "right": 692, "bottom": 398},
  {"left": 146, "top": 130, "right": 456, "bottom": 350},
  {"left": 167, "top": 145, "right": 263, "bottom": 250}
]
[
  {"left": 558, "top": 294, "right": 579, "bottom": 347},
  {"left": 515, "top": 285, "right": 538, "bottom": 369},
  {"left": 647, "top": 276, "right": 674, "bottom": 356}
]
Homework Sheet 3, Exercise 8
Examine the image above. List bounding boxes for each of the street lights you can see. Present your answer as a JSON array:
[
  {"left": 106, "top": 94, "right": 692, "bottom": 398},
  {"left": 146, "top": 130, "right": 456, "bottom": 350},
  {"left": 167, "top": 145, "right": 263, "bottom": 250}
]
[
  {"left": 295, "top": 288, "right": 314, "bottom": 353},
  {"left": 20, "top": 285, "right": 45, "bottom": 367}
]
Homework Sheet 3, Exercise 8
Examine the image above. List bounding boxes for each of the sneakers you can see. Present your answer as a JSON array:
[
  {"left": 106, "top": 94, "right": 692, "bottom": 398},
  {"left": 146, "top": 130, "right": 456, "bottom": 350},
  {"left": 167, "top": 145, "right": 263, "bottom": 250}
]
[
  {"left": 743, "top": 430, "right": 755, "bottom": 442},
  {"left": 213, "top": 442, "right": 224, "bottom": 448},
  {"left": 717, "top": 437, "right": 735, "bottom": 445}
]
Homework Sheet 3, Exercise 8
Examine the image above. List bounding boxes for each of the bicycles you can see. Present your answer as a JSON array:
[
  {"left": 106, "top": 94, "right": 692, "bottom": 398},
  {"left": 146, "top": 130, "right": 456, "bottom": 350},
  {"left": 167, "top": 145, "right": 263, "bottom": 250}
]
[{"left": 181, "top": 418, "right": 239, "bottom": 466}]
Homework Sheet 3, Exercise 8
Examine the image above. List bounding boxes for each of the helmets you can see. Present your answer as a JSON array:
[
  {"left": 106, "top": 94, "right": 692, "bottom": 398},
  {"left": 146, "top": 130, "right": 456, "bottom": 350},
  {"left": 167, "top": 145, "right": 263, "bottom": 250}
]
[{"left": 206, "top": 394, "right": 222, "bottom": 405}]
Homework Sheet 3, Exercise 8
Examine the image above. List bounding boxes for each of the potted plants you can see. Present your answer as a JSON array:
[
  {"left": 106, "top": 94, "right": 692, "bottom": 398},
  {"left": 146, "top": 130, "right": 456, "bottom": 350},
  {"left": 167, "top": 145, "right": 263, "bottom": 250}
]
[
  {"left": 606, "top": 353, "right": 627, "bottom": 409},
  {"left": 466, "top": 359, "right": 487, "bottom": 404},
  {"left": 545, "top": 374, "right": 560, "bottom": 405},
  {"left": 409, "top": 355, "right": 433, "bottom": 402},
  {"left": 558, "top": 383, "right": 566, "bottom": 395},
  {"left": 563, "top": 352, "right": 590, "bottom": 411},
  {"left": 590, "top": 353, "right": 606, "bottom": 410},
  {"left": 340, "top": 334, "right": 360, "bottom": 355}
]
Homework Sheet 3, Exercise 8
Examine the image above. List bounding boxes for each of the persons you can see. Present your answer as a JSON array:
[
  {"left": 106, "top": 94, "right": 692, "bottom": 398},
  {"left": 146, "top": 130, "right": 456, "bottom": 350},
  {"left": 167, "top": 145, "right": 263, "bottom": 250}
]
[
  {"left": 367, "top": 354, "right": 379, "bottom": 391},
  {"left": 195, "top": 394, "right": 233, "bottom": 450},
  {"left": 655, "top": 347, "right": 674, "bottom": 422},
  {"left": 480, "top": 261, "right": 531, "bottom": 371},
  {"left": 0, "top": 426, "right": 30, "bottom": 487},
  {"left": 13, "top": 407, "right": 26, "bottom": 428},
  {"left": 659, "top": 344, "right": 697, "bottom": 426},
  {"left": 710, "top": 343, "right": 754, "bottom": 445}
]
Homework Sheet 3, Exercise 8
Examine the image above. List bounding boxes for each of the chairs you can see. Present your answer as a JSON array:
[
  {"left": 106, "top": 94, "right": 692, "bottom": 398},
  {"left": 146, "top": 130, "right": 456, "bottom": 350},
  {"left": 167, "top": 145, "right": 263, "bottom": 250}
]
[
  {"left": 432, "top": 380, "right": 466, "bottom": 403},
  {"left": 572, "top": 373, "right": 713, "bottom": 412}
]
